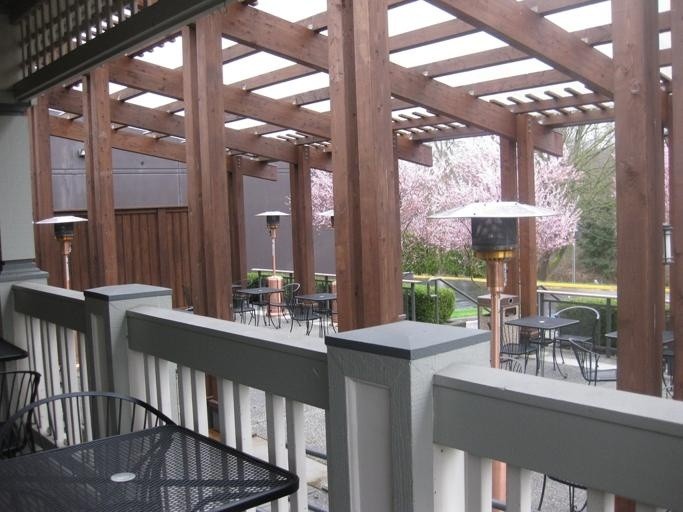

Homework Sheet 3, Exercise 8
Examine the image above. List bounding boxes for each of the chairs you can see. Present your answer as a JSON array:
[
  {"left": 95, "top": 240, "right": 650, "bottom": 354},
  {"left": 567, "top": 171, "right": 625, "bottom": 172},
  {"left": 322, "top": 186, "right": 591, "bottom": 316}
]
[
  {"left": 232, "top": 278, "right": 338, "bottom": 338},
  {"left": 0, "top": 369, "right": 176, "bottom": 458},
  {"left": 486, "top": 304, "right": 675, "bottom": 399}
]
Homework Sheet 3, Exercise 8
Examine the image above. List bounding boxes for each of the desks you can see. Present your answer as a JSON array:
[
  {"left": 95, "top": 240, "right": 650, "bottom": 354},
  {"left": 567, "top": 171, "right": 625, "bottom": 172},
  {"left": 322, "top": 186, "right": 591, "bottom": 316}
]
[{"left": 0, "top": 425, "right": 299, "bottom": 512}]
